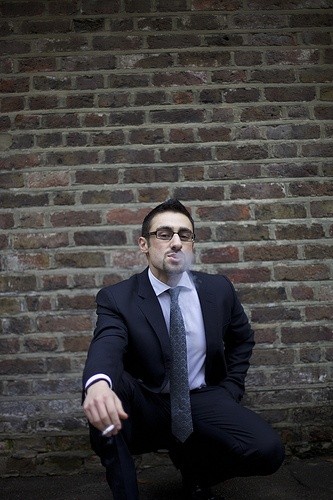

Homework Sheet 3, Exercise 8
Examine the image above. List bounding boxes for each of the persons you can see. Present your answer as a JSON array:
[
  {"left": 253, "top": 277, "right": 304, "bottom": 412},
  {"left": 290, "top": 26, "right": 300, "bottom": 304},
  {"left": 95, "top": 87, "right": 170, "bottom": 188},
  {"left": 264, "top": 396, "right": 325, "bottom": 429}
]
[{"left": 81, "top": 197, "right": 286, "bottom": 500}]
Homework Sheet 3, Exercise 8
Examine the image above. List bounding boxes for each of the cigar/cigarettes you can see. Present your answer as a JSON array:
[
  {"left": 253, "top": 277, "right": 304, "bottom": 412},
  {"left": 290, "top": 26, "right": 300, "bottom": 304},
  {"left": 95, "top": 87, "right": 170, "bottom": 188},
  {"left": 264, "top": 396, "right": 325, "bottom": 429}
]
[{"left": 102, "top": 424, "right": 116, "bottom": 435}]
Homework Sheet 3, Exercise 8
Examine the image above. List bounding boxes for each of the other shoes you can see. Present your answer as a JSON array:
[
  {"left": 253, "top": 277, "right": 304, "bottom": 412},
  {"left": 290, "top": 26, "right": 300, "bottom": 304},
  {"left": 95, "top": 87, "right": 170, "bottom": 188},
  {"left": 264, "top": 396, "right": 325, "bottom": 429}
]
[{"left": 167, "top": 446, "right": 209, "bottom": 498}]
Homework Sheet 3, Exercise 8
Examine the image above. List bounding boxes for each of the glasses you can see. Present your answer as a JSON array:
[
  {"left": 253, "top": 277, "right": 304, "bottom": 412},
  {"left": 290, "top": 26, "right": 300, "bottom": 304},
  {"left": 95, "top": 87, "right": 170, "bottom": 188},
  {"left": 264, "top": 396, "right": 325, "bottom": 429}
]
[{"left": 143, "top": 228, "right": 195, "bottom": 243}]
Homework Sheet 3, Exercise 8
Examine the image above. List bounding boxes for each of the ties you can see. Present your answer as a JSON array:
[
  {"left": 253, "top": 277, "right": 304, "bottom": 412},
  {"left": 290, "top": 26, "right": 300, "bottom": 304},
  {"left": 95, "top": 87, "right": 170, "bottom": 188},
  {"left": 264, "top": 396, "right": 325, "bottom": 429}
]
[{"left": 164, "top": 286, "right": 195, "bottom": 445}]
